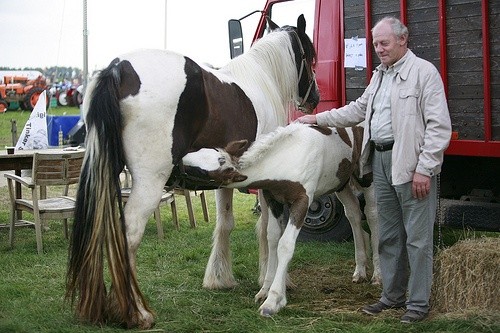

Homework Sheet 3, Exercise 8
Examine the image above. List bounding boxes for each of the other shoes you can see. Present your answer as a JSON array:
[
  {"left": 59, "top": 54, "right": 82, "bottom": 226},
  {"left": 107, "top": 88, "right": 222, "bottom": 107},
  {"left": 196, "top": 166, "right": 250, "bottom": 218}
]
[
  {"left": 400, "top": 309, "right": 427, "bottom": 323},
  {"left": 362, "top": 302, "right": 407, "bottom": 315}
]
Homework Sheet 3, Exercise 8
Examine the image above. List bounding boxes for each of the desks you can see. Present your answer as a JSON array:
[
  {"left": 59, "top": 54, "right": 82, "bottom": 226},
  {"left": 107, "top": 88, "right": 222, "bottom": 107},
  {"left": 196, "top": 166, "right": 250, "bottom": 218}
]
[{"left": 0, "top": 147, "right": 85, "bottom": 231}]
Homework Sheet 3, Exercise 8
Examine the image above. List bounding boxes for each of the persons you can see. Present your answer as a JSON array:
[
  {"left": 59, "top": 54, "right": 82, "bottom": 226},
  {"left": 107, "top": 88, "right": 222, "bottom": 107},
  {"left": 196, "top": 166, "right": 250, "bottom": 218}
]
[{"left": 293, "top": 15, "right": 453, "bottom": 324}]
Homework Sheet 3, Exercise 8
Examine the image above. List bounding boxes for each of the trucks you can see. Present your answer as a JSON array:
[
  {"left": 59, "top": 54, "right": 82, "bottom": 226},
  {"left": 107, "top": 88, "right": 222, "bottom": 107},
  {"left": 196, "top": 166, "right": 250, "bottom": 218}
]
[{"left": 228, "top": 0, "right": 500, "bottom": 240}]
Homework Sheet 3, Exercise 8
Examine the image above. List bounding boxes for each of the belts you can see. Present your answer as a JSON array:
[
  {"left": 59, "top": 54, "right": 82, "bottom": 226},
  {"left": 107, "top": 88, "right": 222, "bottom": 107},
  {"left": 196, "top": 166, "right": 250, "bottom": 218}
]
[{"left": 372, "top": 142, "right": 394, "bottom": 152}]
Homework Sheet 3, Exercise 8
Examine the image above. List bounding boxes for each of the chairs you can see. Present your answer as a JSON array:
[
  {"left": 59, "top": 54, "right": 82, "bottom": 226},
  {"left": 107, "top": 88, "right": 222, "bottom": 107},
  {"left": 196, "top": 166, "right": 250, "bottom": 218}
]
[
  {"left": 119, "top": 170, "right": 209, "bottom": 241},
  {"left": 4, "top": 151, "right": 85, "bottom": 256}
]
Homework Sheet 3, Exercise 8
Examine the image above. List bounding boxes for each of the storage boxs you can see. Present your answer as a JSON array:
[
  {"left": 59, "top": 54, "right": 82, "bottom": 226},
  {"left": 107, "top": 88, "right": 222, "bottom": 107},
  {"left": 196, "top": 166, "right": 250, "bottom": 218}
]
[{"left": 46, "top": 115, "right": 80, "bottom": 146}]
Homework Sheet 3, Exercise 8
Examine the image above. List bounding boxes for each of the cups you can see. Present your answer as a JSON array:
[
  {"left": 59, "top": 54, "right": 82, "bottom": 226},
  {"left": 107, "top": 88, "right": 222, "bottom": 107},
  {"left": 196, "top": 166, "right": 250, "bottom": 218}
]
[{"left": 7, "top": 148, "right": 14, "bottom": 154}]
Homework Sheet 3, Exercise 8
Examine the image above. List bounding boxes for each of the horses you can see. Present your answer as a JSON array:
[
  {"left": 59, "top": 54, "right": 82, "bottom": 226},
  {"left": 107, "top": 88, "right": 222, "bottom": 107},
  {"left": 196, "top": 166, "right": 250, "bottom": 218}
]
[{"left": 63, "top": 14, "right": 384, "bottom": 330}]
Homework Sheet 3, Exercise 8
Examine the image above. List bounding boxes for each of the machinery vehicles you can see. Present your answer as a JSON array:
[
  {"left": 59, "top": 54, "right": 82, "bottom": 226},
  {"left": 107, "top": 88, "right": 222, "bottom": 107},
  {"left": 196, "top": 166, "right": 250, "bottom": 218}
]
[{"left": 0, "top": 74, "right": 53, "bottom": 112}]
